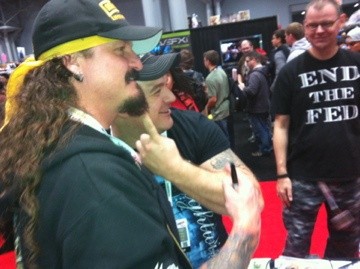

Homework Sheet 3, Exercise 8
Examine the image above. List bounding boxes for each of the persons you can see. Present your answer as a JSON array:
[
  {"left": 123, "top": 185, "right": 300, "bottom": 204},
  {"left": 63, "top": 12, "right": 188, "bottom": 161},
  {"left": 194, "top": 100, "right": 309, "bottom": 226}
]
[{"left": 0, "top": 0, "right": 360, "bottom": 269}]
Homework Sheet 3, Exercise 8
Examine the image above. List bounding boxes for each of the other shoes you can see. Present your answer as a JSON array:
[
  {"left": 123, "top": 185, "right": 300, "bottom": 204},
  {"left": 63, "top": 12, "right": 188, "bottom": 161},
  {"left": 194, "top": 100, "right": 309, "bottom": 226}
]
[
  {"left": 248, "top": 133, "right": 255, "bottom": 143},
  {"left": 251, "top": 150, "right": 263, "bottom": 157}
]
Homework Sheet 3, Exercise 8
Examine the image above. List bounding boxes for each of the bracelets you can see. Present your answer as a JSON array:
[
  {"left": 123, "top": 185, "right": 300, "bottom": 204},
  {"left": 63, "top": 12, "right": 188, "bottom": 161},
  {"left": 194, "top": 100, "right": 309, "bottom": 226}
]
[{"left": 277, "top": 173, "right": 289, "bottom": 179}]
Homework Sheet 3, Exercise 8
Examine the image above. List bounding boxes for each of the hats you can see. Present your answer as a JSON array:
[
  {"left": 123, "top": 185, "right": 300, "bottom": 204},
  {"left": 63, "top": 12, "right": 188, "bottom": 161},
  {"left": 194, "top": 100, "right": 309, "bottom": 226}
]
[
  {"left": 32, "top": 0, "right": 164, "bottom": 61},
  {"left": 133, "top": 53, "right": 181, "bottom": 80}
]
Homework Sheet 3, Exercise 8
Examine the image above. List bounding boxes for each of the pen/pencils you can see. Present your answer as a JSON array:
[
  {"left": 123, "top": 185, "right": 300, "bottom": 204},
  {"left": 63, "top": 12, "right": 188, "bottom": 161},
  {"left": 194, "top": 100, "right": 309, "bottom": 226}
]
[{"left": 231, "top": 161, "right": 239, "bottom": 189}]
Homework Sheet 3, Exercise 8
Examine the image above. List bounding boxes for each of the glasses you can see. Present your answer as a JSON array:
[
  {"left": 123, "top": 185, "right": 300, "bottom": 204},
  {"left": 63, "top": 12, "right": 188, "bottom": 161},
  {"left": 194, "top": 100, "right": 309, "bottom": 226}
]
[{"left": 306, "top": 18, "right": 340, "bottom": 28}]
